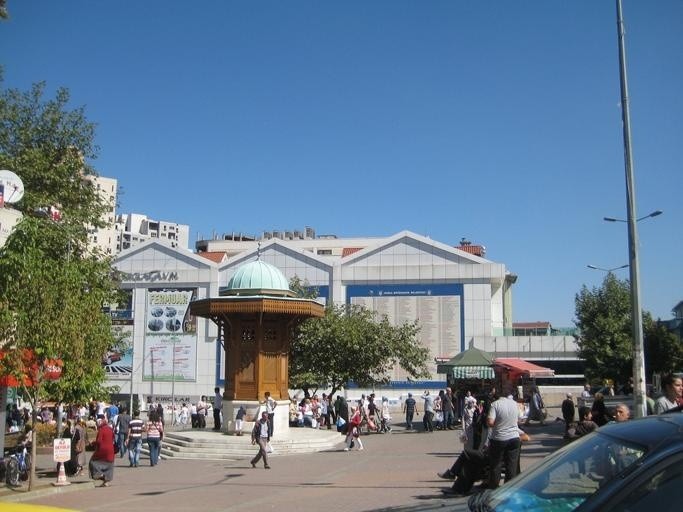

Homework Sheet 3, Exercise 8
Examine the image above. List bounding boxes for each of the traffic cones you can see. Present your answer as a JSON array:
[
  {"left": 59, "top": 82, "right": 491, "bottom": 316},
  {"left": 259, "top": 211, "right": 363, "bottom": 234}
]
[{"left": 52, "top": 462, "right": 73, "bottom": 488}]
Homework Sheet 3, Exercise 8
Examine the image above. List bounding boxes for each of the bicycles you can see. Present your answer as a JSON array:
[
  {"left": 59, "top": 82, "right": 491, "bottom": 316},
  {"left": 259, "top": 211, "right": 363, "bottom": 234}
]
[
  {"left": 5, "top": 444, "right": 30, "bottom": 486},
  {"left": 361, "top": 411, "right": 393, "bottom": 436}
]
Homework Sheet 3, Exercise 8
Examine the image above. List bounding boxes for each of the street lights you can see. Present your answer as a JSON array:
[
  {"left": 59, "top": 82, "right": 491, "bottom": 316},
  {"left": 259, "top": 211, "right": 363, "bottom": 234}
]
[
  {"left": 583, "top": 260, "right": 627, "bottom": 286},
  {"left": 603, "top": 211, "right": 661, "bottom": 417}
]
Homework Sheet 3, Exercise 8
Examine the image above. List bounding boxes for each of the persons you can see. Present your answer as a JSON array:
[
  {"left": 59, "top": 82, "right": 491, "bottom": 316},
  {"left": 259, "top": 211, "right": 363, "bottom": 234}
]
[
  {"left": 5, "top": 387, "right": 222, "bottom": 487},
  {"left": 290, "top": 393, "right": 393, "bottom": 451},
  {"left": 404, "top": 372, "right": 683, "bottom": 496},
  {"left": 234, "top": 405, "right": 247, "bottom": 436},
  {"left": 250, "top": 411, "right": 271, "bottom": 469},
  {"left": 260, "top": 392, "right": 277, "bottom": 437}
]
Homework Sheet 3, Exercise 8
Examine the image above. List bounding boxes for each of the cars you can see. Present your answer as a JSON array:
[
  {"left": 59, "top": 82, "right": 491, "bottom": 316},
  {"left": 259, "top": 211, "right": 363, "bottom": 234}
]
[{"left": 469, "top": 412, "right": 683, "bottom": 511}]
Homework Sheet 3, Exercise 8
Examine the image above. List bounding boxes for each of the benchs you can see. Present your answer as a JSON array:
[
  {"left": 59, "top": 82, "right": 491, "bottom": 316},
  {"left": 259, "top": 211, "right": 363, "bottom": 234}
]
[{"left": 485, "top": 441, "right": 521, "bottom": 482}]
